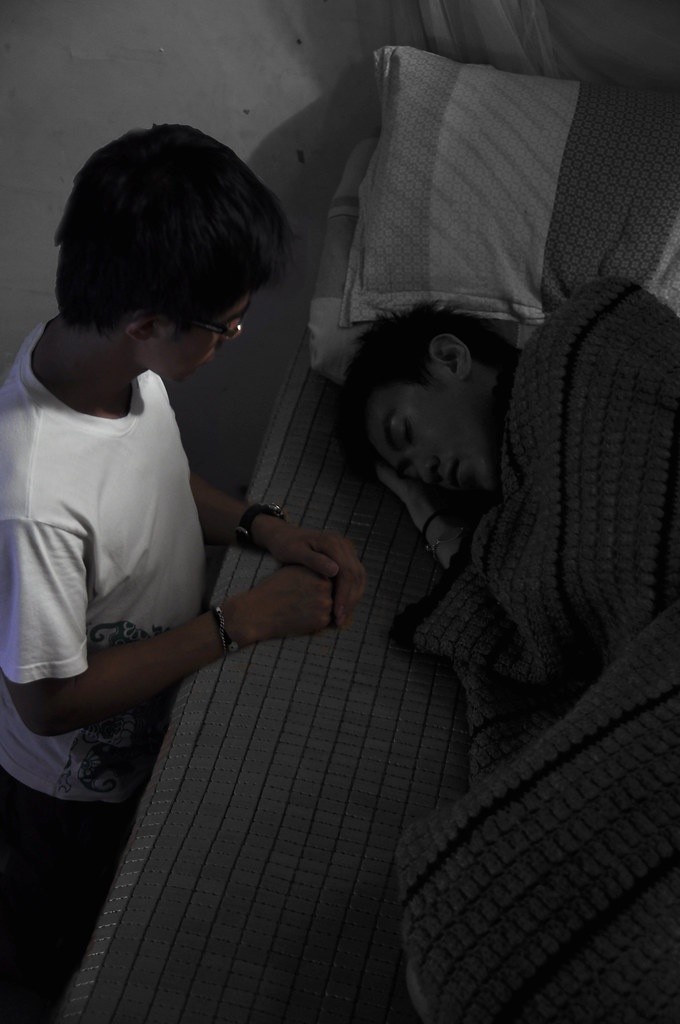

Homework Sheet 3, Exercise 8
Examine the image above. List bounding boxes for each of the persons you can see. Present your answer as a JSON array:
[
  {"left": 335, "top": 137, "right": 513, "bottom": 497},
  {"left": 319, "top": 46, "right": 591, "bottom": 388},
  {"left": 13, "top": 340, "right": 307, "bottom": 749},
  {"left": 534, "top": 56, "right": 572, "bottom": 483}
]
[
  {"left": 334, "top": 275, "right": 679, "bottom": 1023},
  {"left": 0, "top": 123, "right": 372, "bottom": 999}
]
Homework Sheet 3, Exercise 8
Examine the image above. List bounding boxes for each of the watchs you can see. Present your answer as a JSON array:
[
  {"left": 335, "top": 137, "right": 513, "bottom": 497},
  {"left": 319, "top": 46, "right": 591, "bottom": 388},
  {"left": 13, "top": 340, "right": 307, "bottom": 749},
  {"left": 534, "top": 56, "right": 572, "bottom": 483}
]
[{"left": 235, "top": 502, "right": 285, "bottom": 548}]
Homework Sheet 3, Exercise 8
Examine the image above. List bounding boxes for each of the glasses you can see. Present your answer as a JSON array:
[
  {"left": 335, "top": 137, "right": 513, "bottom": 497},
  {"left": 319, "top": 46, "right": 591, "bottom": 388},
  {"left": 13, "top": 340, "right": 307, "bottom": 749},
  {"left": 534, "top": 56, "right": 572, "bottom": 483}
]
[{"left": 188, "top": 300, "right": 251, "bottom": 338}]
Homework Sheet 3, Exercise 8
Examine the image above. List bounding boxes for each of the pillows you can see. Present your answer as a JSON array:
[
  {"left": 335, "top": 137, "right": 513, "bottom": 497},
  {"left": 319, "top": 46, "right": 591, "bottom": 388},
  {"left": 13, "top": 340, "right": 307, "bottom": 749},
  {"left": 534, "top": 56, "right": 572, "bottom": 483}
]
[{"left": 339, "top": 46, "right": 680, "bottom": 328}]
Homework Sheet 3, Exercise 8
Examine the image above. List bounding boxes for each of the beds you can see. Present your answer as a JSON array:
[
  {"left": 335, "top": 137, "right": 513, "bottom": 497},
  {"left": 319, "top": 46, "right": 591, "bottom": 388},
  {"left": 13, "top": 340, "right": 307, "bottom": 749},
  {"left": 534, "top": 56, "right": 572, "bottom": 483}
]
[{"left": 59, "top": 0, "right": 680, "bottom": 1024}]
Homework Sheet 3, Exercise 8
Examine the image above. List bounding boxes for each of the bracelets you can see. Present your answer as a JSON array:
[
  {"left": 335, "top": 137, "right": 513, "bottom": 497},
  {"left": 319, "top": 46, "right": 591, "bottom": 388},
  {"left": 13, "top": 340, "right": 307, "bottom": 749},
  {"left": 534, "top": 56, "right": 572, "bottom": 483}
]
[
  {"left": 427, "top": 525, "right": 464, "bottom": 559},
  {"left": 210, "top": 605, "right": 240, "bottom": 653},
  {"left": 421, "top": 510, "right": 440, "bottom": 542},
  {"left": 215, "top": 606, "right": 227, "bottom": 652}
]
